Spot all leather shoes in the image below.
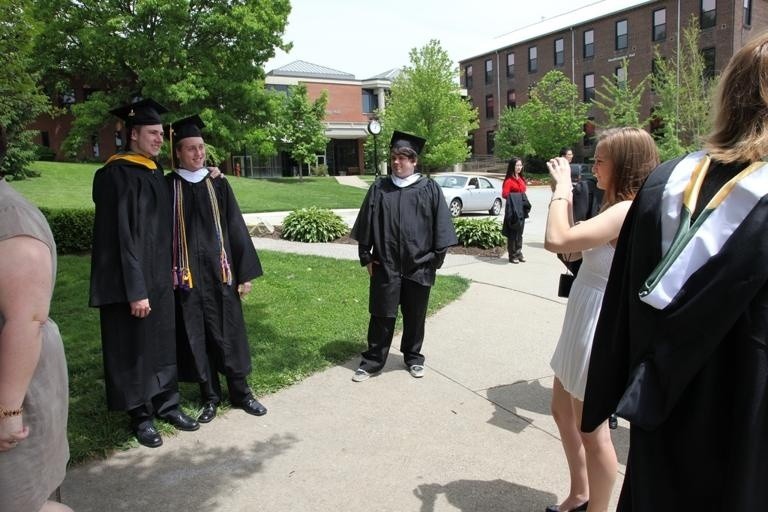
[546,501,588,512]
[136,422,161,447]
[199,403,219,421]
[165,409,199,430]
[243,397,267,416]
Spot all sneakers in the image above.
[352,367,382,382]
[409,364,424,378]
[509,258,525,263]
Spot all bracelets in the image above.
[0,407,23,418]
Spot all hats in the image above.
[108,98,169,126]
[162,114,207,172]
[387,130,426,155]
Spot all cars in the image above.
[426,171,504,219]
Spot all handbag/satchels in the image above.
[557,275,575,297]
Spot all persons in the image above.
[579,30,767,512]
[349,130,458,383]
[502,157,531,263]
[164,113,268,423]
[542,128,660,511]
[89,96,225,448]
[0,127,78,512]
